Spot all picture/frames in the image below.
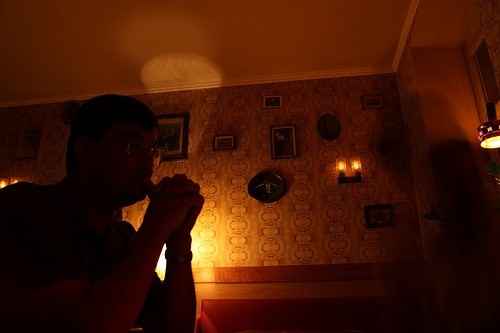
[361,93,384,109]
[263,94,282,109]
[213,133,236,151]
[14,123,47,166]
[364,203,395,230]
[270,124,298,160]
[153,112,190,162]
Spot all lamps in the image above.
[336,156,364,185]
[476,119,500,149]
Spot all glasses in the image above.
[104,136,160,158]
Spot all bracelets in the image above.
[163,249,193,264]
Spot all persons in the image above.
[1,94,204,332]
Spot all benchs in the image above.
[194,297,441,333]
